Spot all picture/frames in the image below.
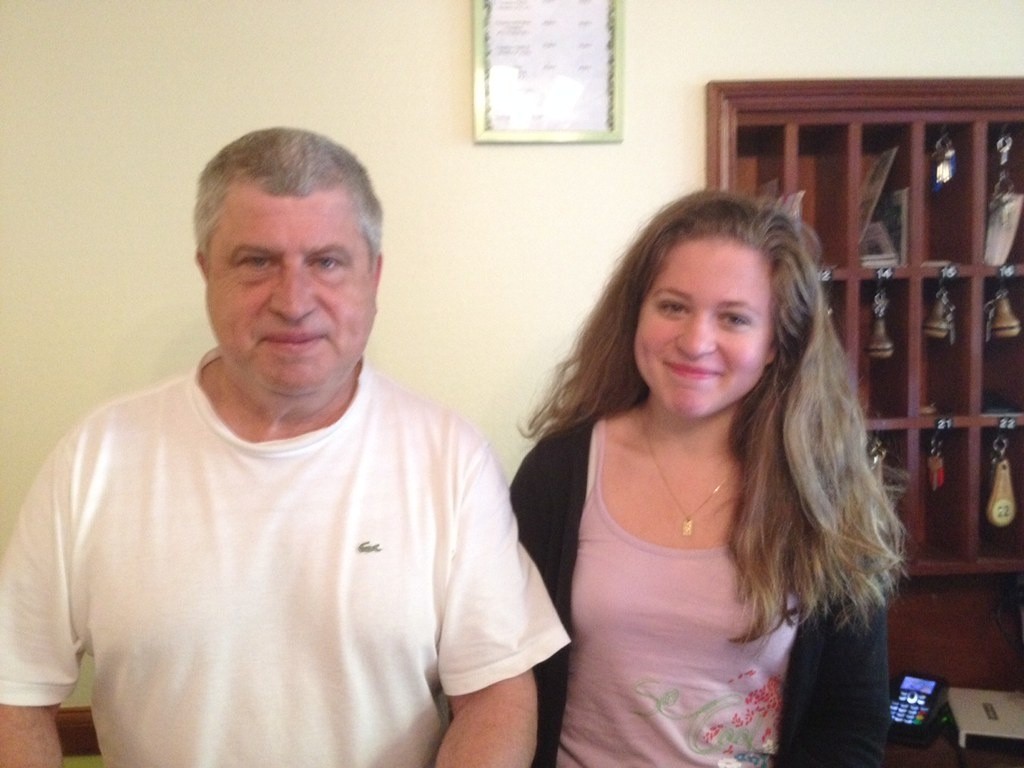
[471,0,627,142]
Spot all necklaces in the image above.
[640,413,735,539]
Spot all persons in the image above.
[0,128,572,768]
[506,188,910,768]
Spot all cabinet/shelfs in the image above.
[706,77,1023,583]
